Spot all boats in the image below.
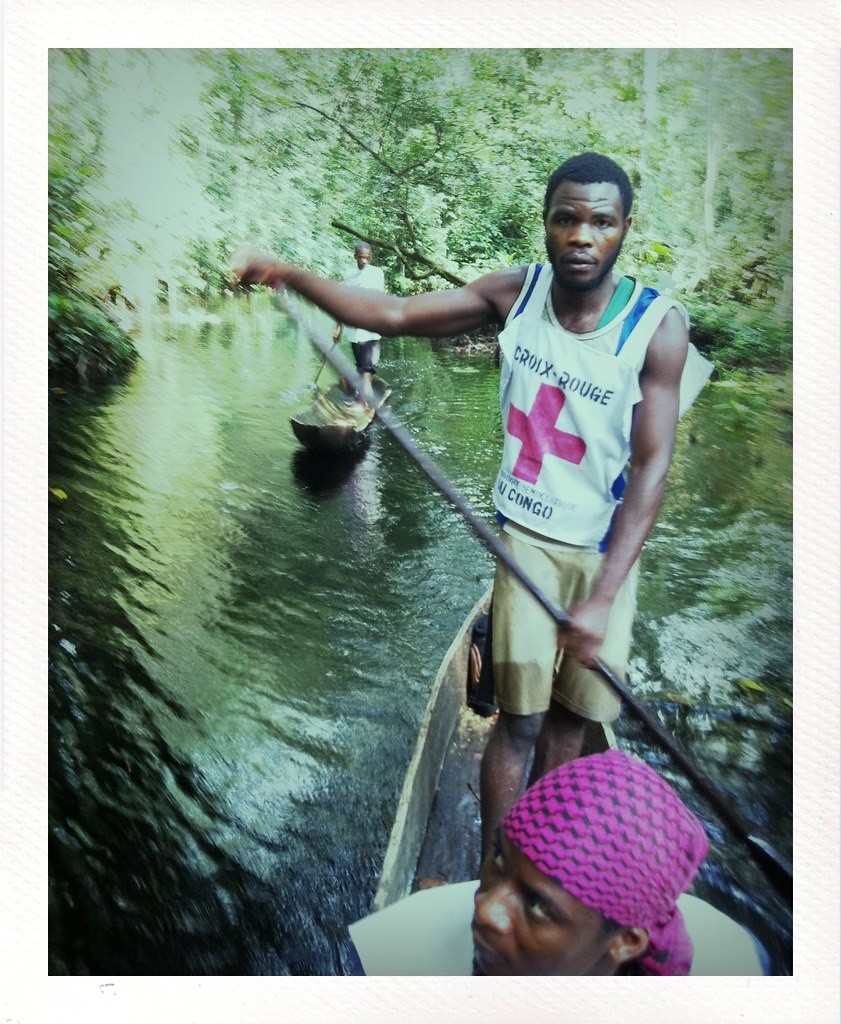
[289,371,397,458]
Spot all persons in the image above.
[348,750,772,976]
[235,152,688,884]
[331,242,384,411]
